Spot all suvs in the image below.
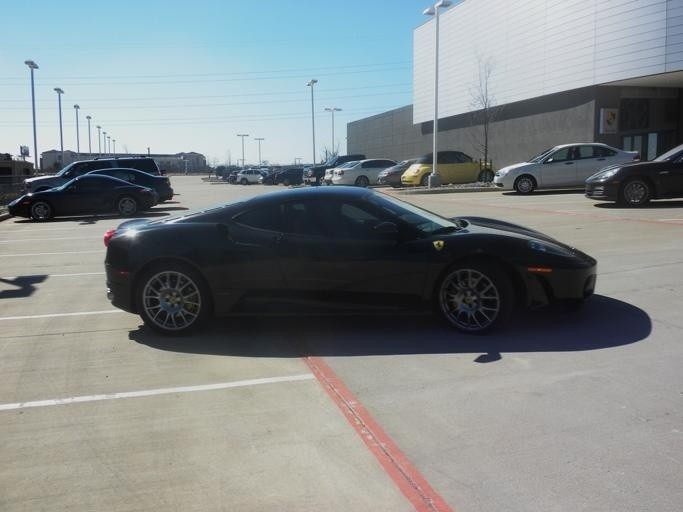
[21,154,161,196]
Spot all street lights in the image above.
[22,59,116,179]
[236,133,264,169]
[304,78,318,164]
[324,107,342,157]
[422,0,452,178]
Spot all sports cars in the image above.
[583,141,683,208]
[98,185,599,335]
[6,172,158,221]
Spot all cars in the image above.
[492,141,640,195]
[86,167,172,204]
[227,151,498,190]
